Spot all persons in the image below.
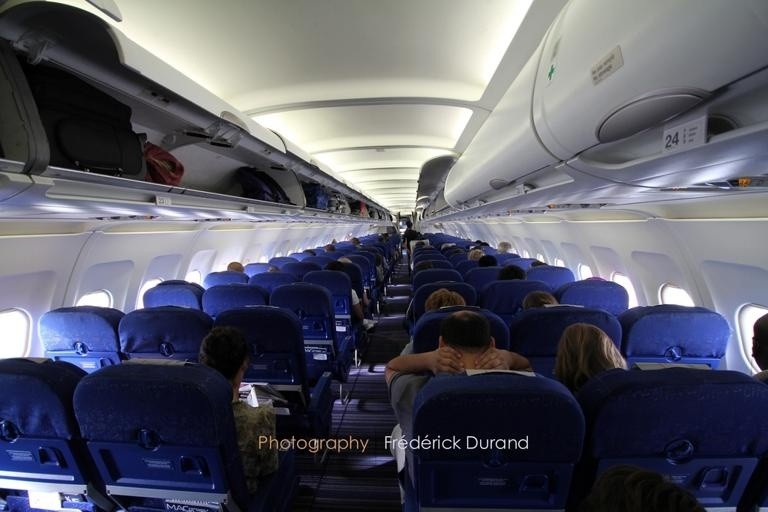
[553,322,627,392]
[196,218,625,354]
[382,310,533,504]
[750,311,768,384]
[198,327,279,495]
[568,462,708,511]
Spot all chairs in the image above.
[2,232,768,512]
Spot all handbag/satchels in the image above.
[301,181,385,220]
[18,54,183,186]
[237,167,290,203]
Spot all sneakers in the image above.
[363,318,378,330]
